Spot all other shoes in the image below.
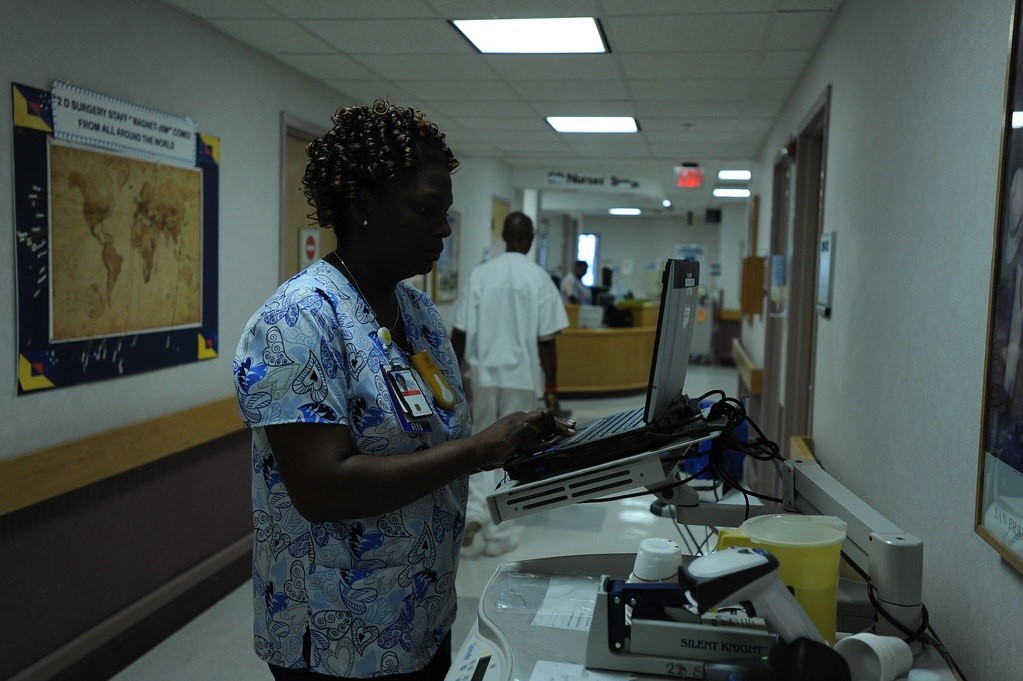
[484,531,520,556]
[461,517,482,547]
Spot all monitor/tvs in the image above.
[598,294,615,323]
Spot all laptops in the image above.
[504,258,701,481]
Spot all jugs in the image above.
[712,514,846,646]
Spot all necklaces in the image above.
[333,249,399,331]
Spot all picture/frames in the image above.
[973,0,1023,576]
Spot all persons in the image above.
[559,261,593,305]
[450,211,571,557]
[395,373,410,392]
[233,98,577,681]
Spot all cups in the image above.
[833,633,913,681]
[629,538,682,583]
